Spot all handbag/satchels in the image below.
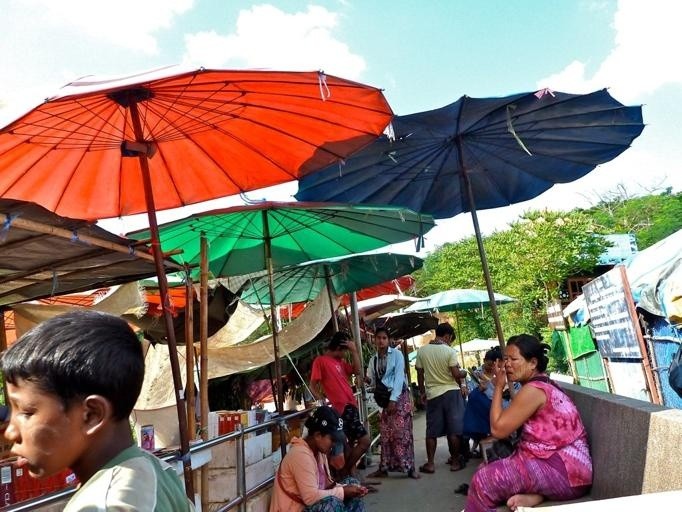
[373,381,391,409]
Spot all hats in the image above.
[304,405,348,443]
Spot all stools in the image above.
[479,438,494,464]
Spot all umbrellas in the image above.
[238,248,426,306]
[294,84,649,401]
[272,272,416,320]
[126,199,438,458]
[341,294,431,324]
[0,61,398,505]
[371,313,440,386]
[404,289,519,385]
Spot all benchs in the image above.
[533,379,682,510]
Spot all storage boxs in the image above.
[197,431,277,512]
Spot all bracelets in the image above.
[461,383,466,388]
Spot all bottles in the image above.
[351,374,356,385]
[216,411,248,436]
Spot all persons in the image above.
[308,329,380,494]
[462,349,496,440]
[0,307,205,512]
[362,328,420,480]
[267,404,369,512]
[462,332,594,512]
[414,322,468,473]
[479,347,523,411]
[447,332,471,463]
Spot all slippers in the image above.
[419,462,435,474]
[367,470,389,478]
[408,471,421,480]
[450,461,466,471]
